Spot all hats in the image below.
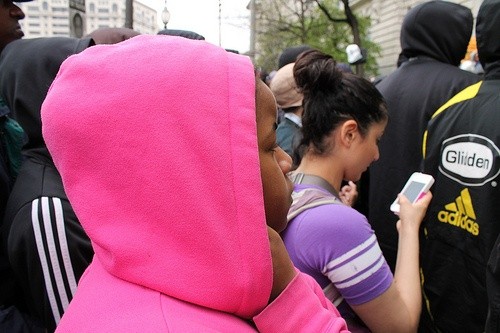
[345,43,363,64]
[270,63,304,109]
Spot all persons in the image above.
[39,33,351,333]
[352,0,481,280]
[421,0,500,333]
[279,49,433,333]
[0,0,484,332]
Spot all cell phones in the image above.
[390,172,435,214]
